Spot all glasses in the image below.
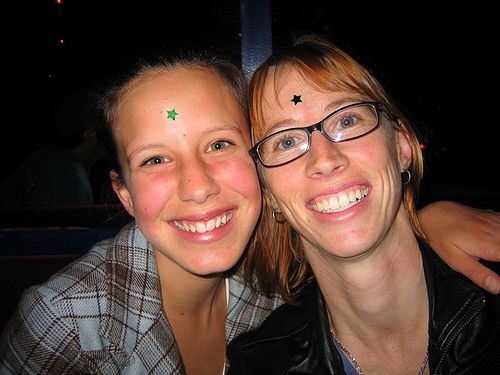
[247,102,398,168]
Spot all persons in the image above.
[0,43,500,375]
[214,35,500,375]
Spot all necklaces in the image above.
[331,330,427,374]
